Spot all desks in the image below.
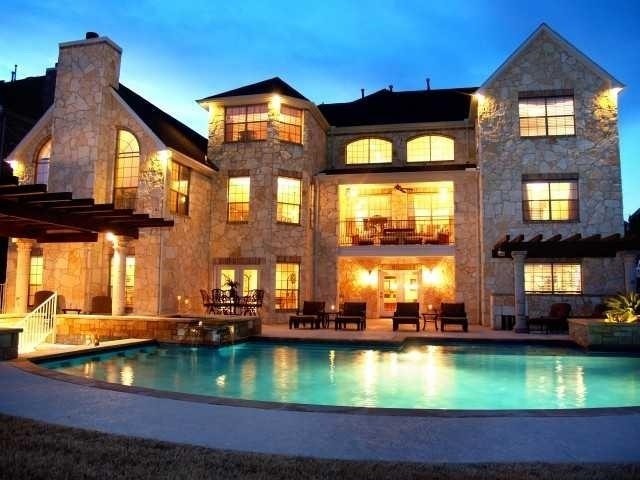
[61,308,81,314]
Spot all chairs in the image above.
[33,290,54,311]
[288,300,367,331]
[351,228,450,245]
[91,295,112,314]
[525,303,611,335]
[391,302,468,333]
[199,288,264,316]
